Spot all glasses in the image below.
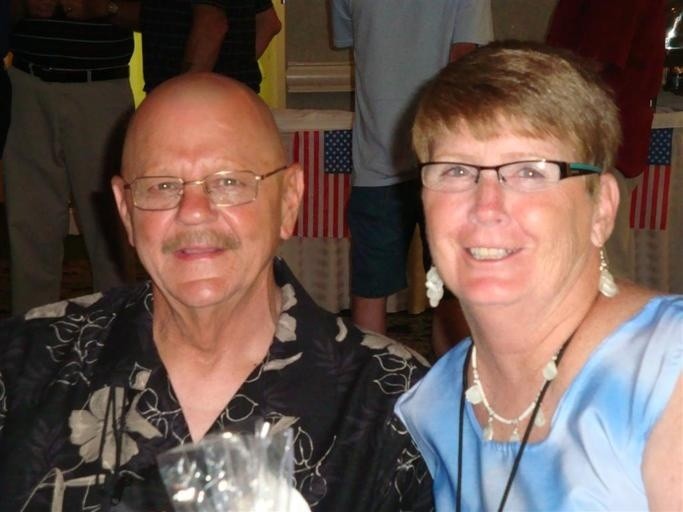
[416,159,602,190]
[124,165,287,212]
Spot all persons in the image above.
[392,39,682,509]
[0,1,140,310]
[1,69,434,511]
[327,1,499,363]
[539,2,669,285]
[136,1,283,99]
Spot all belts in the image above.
[11,60,130,83]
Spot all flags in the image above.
[627,128,672,229]
[280,129,352,240]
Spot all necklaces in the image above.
[465,346,556,445]
